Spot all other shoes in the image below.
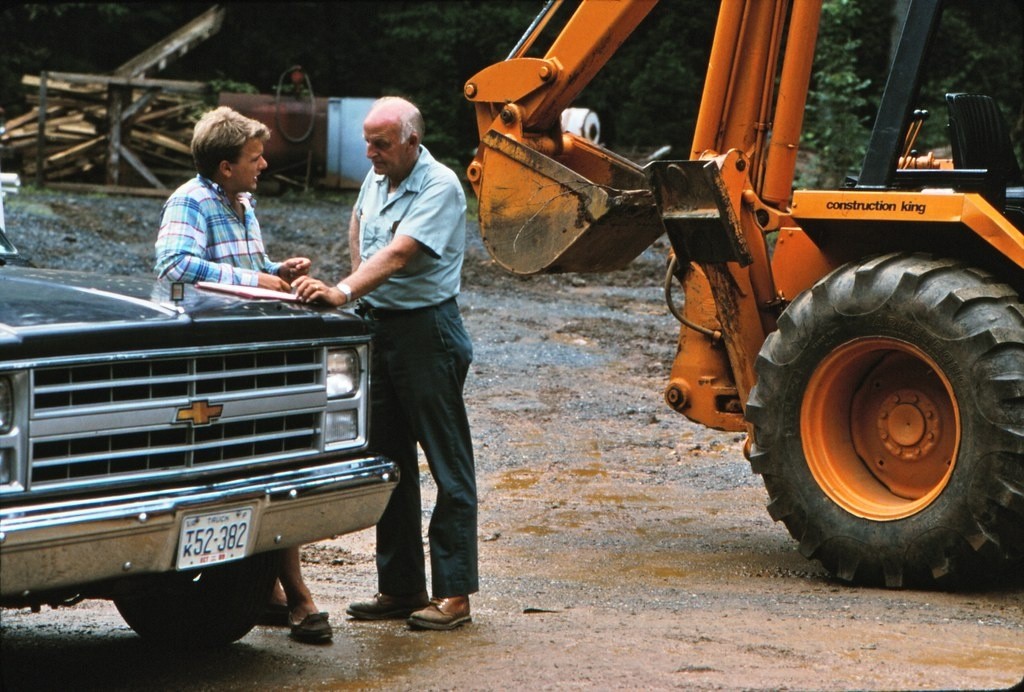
[286,609,333,641]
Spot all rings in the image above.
[314,283,319,291]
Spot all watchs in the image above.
[338,283,351,304]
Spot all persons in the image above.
[154,106,333,641]
[291,96,480,630]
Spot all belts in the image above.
[365,308,395,320]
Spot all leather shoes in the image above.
[346,592,430,619]
[408,595,472,629]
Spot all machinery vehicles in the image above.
[461,0,1024,591]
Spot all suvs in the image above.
[0,220,402,651]
